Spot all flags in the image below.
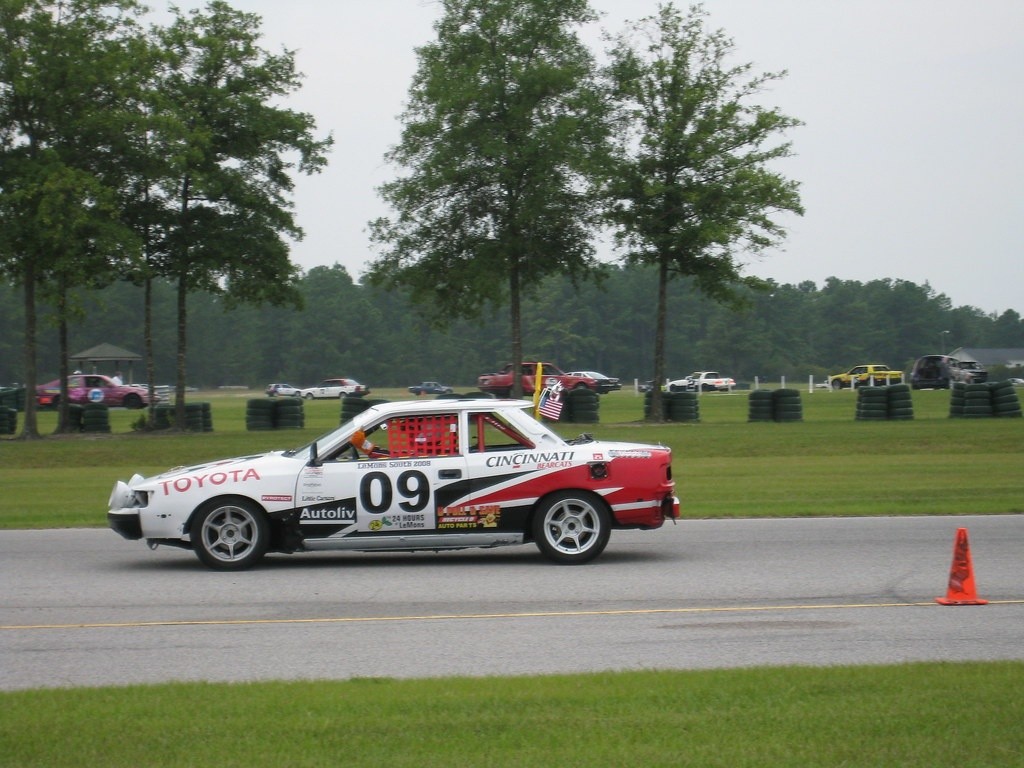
[537,396,563,420]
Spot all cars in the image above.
[478,362,601,398]
[688,371,737,392]
[300,378,374,396]
[909,353,989,391]
[565,370,623,395]
[409,382,455,396]
[830,365,904,390]
[266,383,303,398]
[35,374,149,411]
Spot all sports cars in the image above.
[105,395,680,571]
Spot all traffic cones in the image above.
[936,527,992,606]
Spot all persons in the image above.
[350,418,444,457]
[112,371,123,385]
